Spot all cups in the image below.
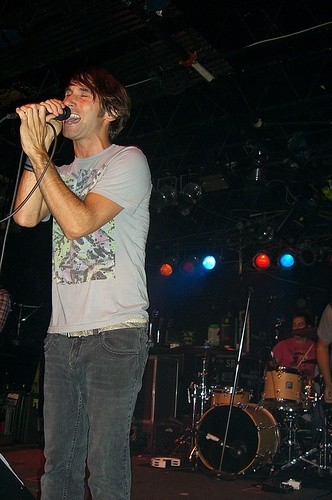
[154,328,162,344]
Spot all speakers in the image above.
[131,355,180,425]
[0,453,37,500]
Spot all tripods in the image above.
[160,416,236,480]
[267,397,332,483]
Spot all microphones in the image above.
[234,447,247,456]
[271,351,278,367]
[8,106,71,121]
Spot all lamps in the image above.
[152,175,176,207]
[179,182,202,204]
[252,250,272,271]
[277,251,297,271]
[216,136,289,188]
[180,255,198,275]
[159,248,177,276]
[200,247,223,270]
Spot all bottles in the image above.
[220,318,233,346]
[208,324,220,346]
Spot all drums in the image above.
[261,365,305,405]
[194,402,281,480]
[210,386,250,408]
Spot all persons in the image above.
[0,288,11,330]
[13,66,152,500]
[267,308,317,385]
[317,299,332,404]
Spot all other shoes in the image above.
[282,479,300,490]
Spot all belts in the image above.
[59,319,147,337]
